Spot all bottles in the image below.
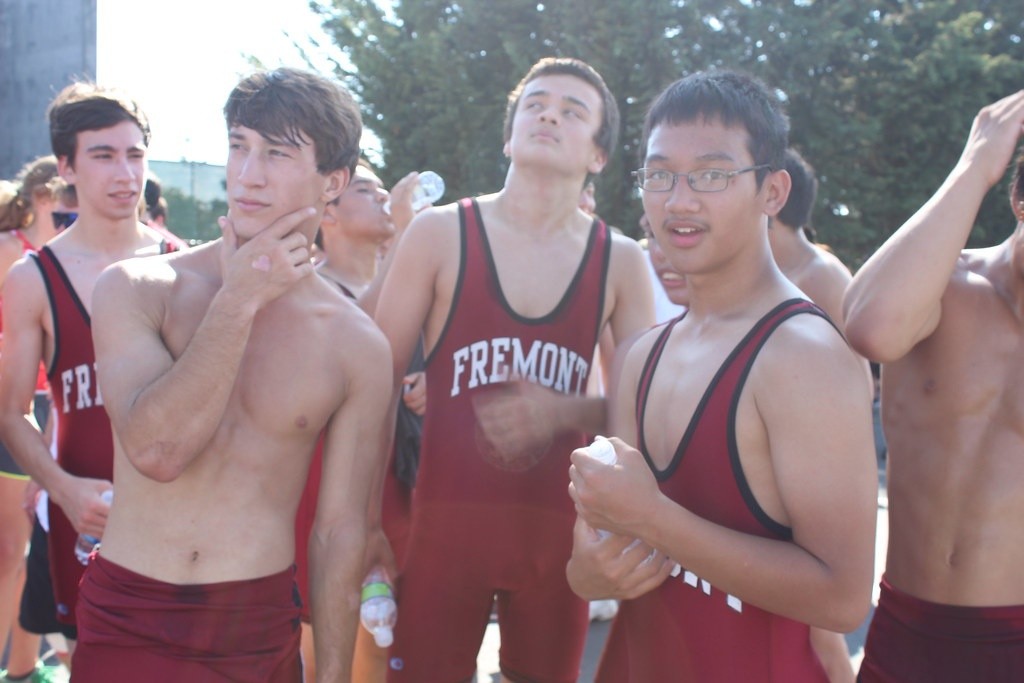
[359,563,397,647]
[384,171,446,216]
[74,487,116,565]
[573,438,672,571]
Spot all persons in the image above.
[574,180,620,396]
[766,147,877,406]
[0,180,26,232]
[0,155,68,683]
[292,155,435,683]
[137,170,831,329]
[564,71,880,683]
[840,87,1024,683]
[368,57,658,683]
[634,202,858,682]
[0,81,183,628]
[68,68,394,682]
[19,176,88,683]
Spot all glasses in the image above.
[631,162,773,192]
